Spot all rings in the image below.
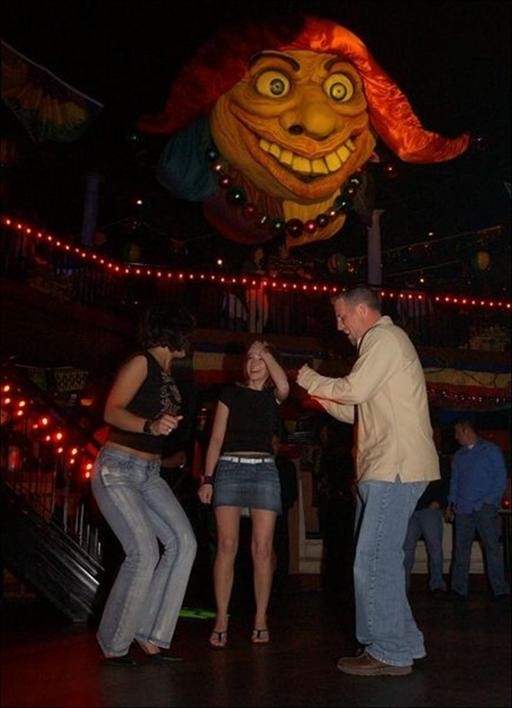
[166,427,172,431]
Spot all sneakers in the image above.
[428,584,468,602]
[335,647,413,677]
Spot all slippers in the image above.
[251,624,269,645]
[208,629,228,651]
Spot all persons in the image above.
[293,284,441,676]
[10,212,57,288]
[159,429,202,608]
[195,334,290,651]
[269,240,314,333]
[443,414,511,605]
[240,243,270,333]
[87,300,202,671]
[78,227,119,307]
[270,428,299,595]
[403,418,449,598]
[307,417,355,600]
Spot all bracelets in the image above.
[200,474,213,485]
[143,419,153,436]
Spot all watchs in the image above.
[259,345,271,357]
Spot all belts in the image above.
[219,455,275,465]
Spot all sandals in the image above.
[130,627,183,662]
[102,654,146,678]
